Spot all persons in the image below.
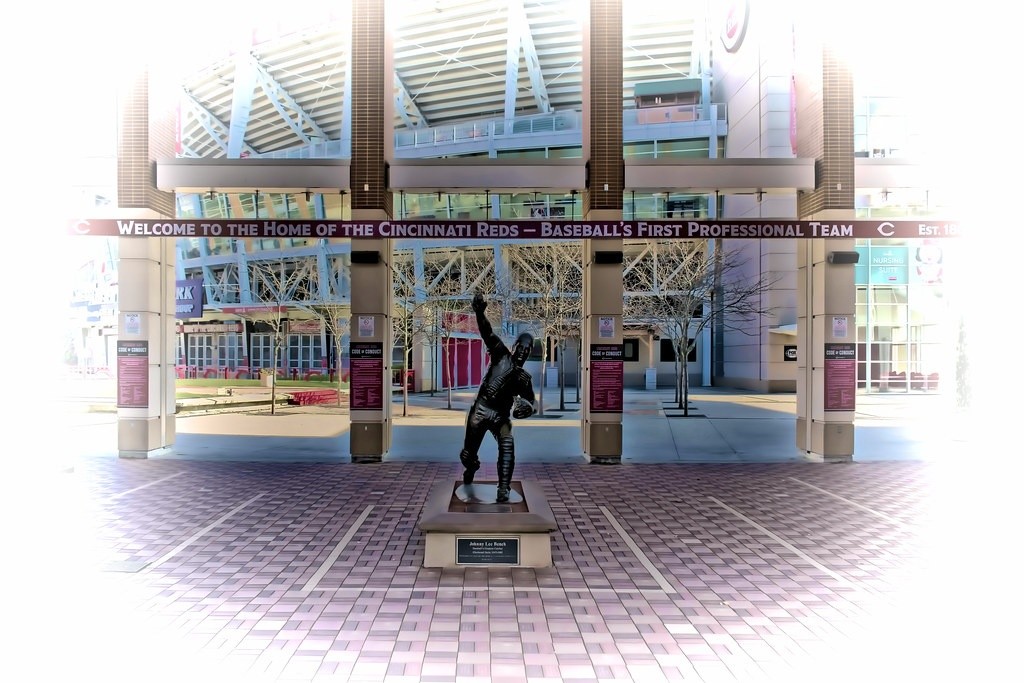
[460,293,538,501]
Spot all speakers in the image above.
[829,252,859,264]
[595,251,623,264]
[350,251,379,263]
